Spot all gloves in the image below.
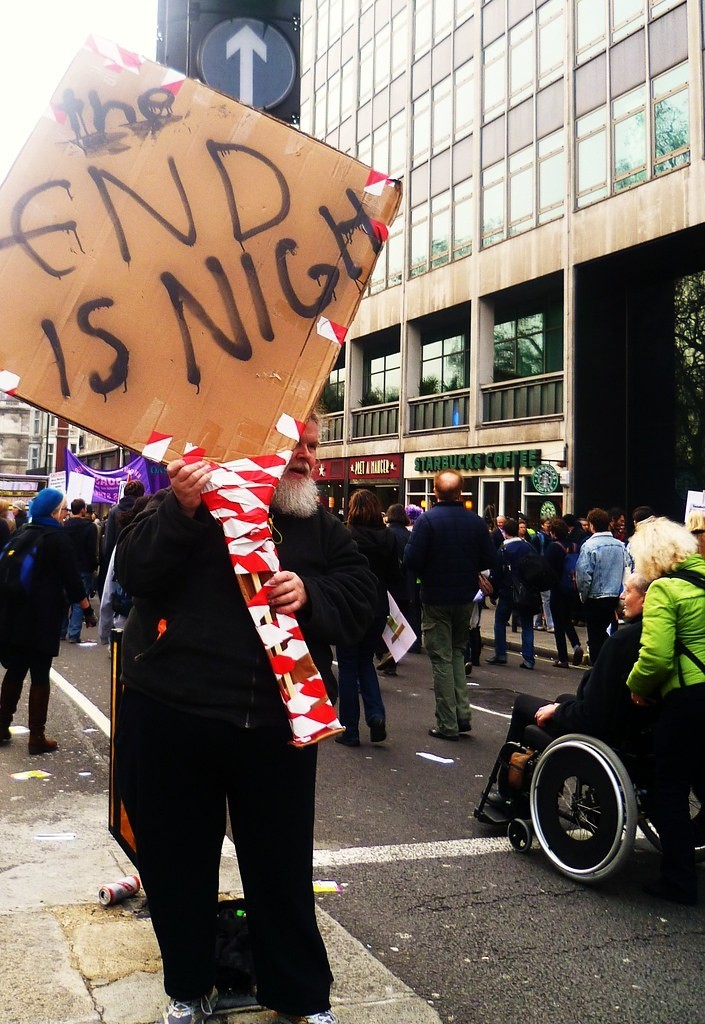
[83,605,97,628]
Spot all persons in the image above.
[400,469,500,736]
[113,400,390,1024]
[510,569,661,793]
[0,479,154,757]
[460,504,653,675]
[316,485,425,749]
[626,516,704,854]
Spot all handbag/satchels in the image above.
[512,583,542,611]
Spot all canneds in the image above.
[99,875,141,905]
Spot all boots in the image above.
[0,681,22,741]
[29,685,57,755]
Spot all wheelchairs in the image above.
[473,690,705,887]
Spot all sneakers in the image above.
[163,997,213,1024]
[278,1009,339,1024]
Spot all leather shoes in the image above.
[482,787,505,806]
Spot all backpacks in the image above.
[0,529,53,601]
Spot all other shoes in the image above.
[458,720,472,732]
[335,734,359,746]
[370,717,386,742]
[376,652,394,670]
[553,660,569,668]
[428,728,458,740]
[465,661,472,675]
[641,870,698,904]
[573,647,584,666]
[70,638,81,642]
[485,657,506,663]
[520,662,527,668]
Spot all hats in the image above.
[29,488,63,516]
